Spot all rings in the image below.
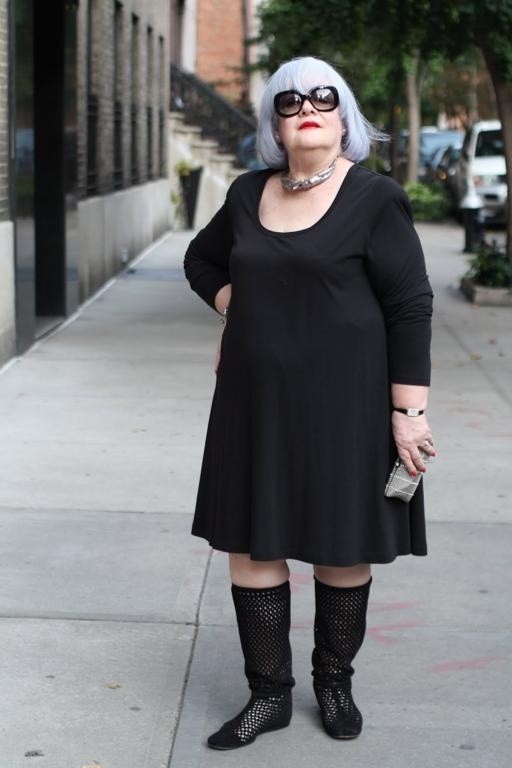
[421,439,430,450]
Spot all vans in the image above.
[450,118,509,229]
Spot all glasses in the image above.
[273,87,337,118]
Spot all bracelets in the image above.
[221,307,228,322]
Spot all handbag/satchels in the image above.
[385,448,429,504]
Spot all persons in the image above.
[183,55,435,749]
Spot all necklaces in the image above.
[278,159,338,190]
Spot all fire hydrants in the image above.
[458,194,487,251]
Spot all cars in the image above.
[425,146,464,199]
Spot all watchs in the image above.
[396,408,425,416]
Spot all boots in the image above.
[208,582,296,750]
[313,580,372,741]
